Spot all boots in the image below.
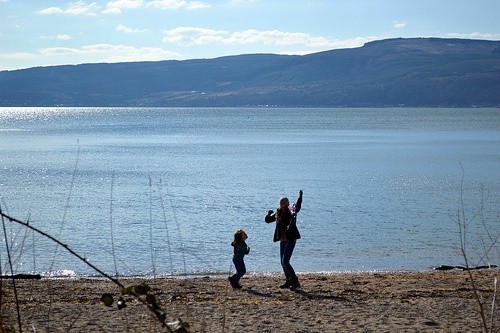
[290,276,300,290]
[280,279,292,288]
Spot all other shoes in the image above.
[235,284,242,289]
[228,277,235,288]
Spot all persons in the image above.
[228,229,250,290]
[265,189,304,291]
